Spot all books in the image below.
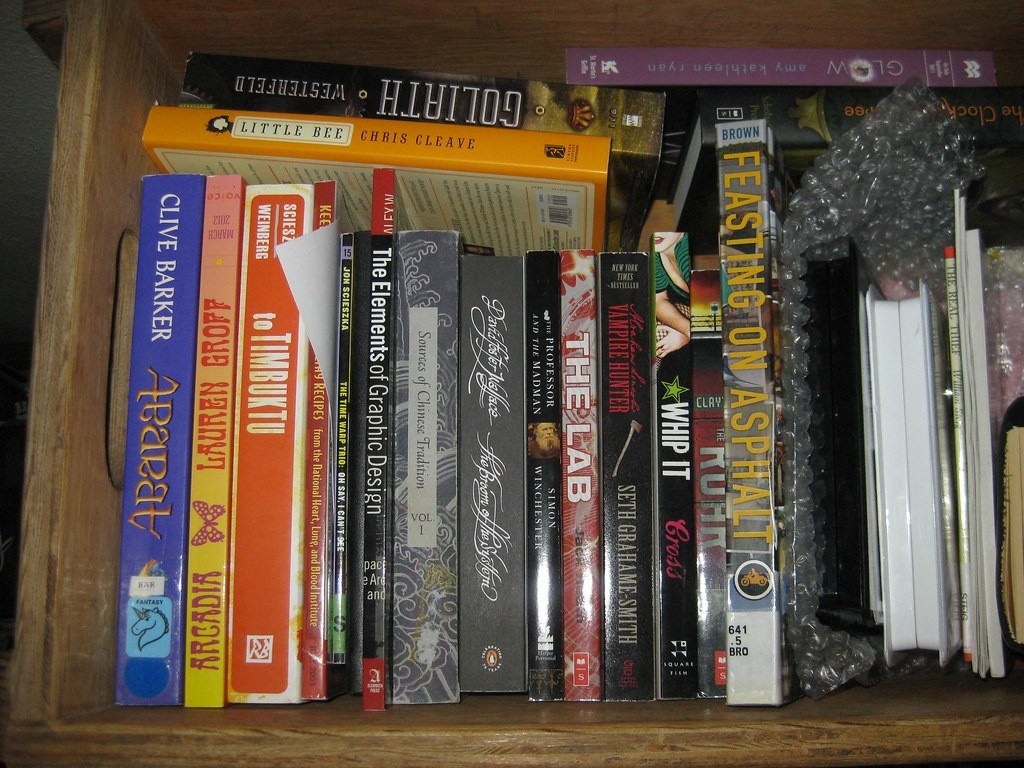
[115,47,1024,709]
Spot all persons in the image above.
[527,422,560,459]
[653,232,691,358]
